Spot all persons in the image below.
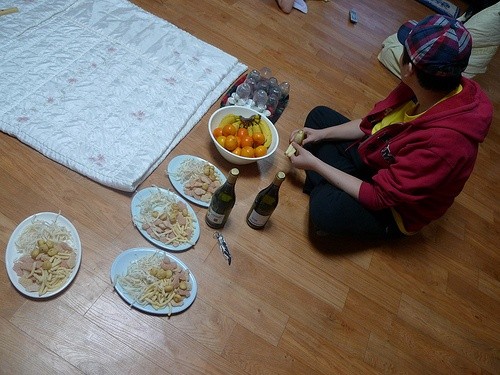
[289,14,493,256]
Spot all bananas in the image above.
[284,130,305,157]
[219,114,272,149]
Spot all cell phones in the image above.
[350,9,357,22]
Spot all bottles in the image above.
[225,69,291,118]
[205,168,239,229]
[246,171,286,230]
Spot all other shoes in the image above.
[277,0,294,14]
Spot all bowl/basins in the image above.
[208,105,279,165]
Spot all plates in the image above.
[111,247,198,315]
[130,188,200,251]
[5,211,81,298]
[167,154,226,208]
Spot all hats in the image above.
[397,15,473,77]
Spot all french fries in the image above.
[27,251,72,296]
[177,159,207,184]
[145,209,197,245]
[140,277,177,317]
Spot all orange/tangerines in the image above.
[213,125,267,158]
